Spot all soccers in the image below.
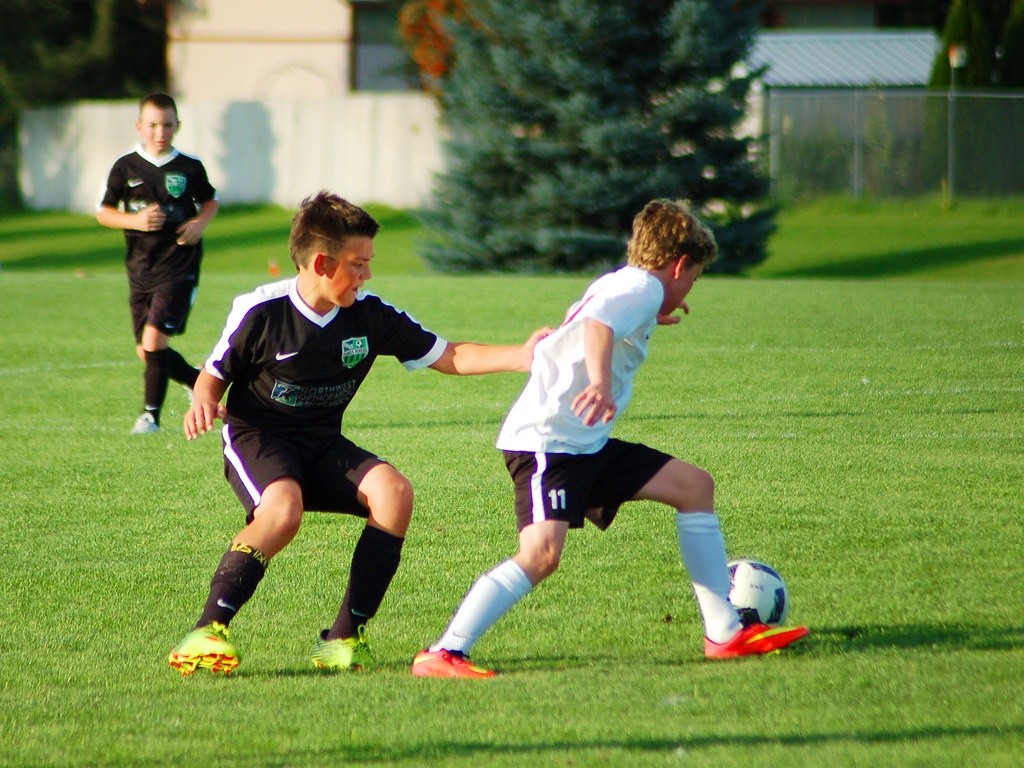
[726,559,788,626]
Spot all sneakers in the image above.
[310,629,380,673]
[169,621,239,677]
[412,648,497,679]
[704,623,809,659]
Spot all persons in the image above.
[411,198,810,679]
[165,190,557,677]
[94,92,222,434]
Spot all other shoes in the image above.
[131,415,161,434]
[185,366,205,407]
